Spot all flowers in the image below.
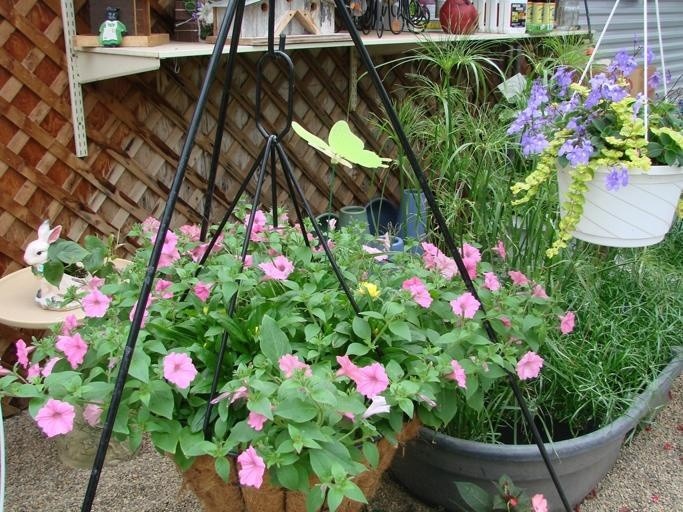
[197,4,215,26]
[1,199,573,512]
[501,42,683,256]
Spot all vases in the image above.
[310,189,425,252]
[164,412,418,512]
[551,149,683,249]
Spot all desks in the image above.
[0,259,147,468]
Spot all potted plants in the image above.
[388,20,683,508]
